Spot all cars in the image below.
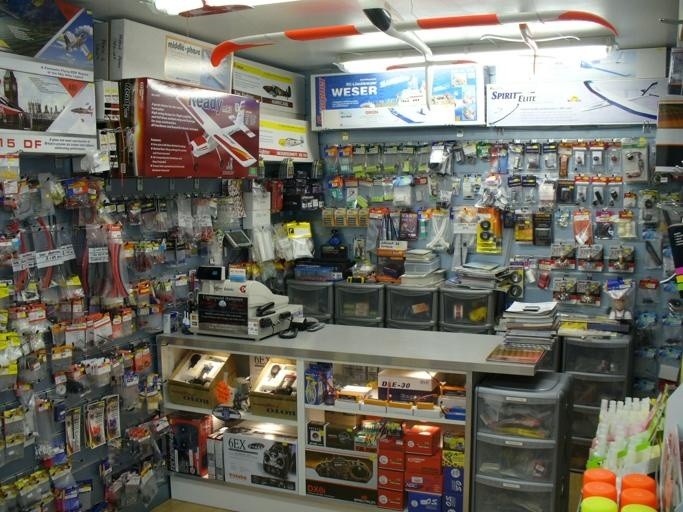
[0,95,25,125]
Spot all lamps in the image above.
[141,0,254,18]
[333,34,615,77]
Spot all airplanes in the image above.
[263,83,293,101]
[60,26,92,60]
[176,93,257,172]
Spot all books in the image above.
[486,299,558,364]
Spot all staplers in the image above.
[256,302,275,317]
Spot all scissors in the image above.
[186,287,199,311]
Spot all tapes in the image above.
[510,271,522,297]
[55,385,66,395]
[480,220,490,240]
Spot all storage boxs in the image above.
[561,334,633,471]
[441,279,494,328]
[472,372,577,510]
[306,361,464,512]
[387,280,439,326]
[335,278,383,322]
[161,340,296,496]
[287,279,332,319]
